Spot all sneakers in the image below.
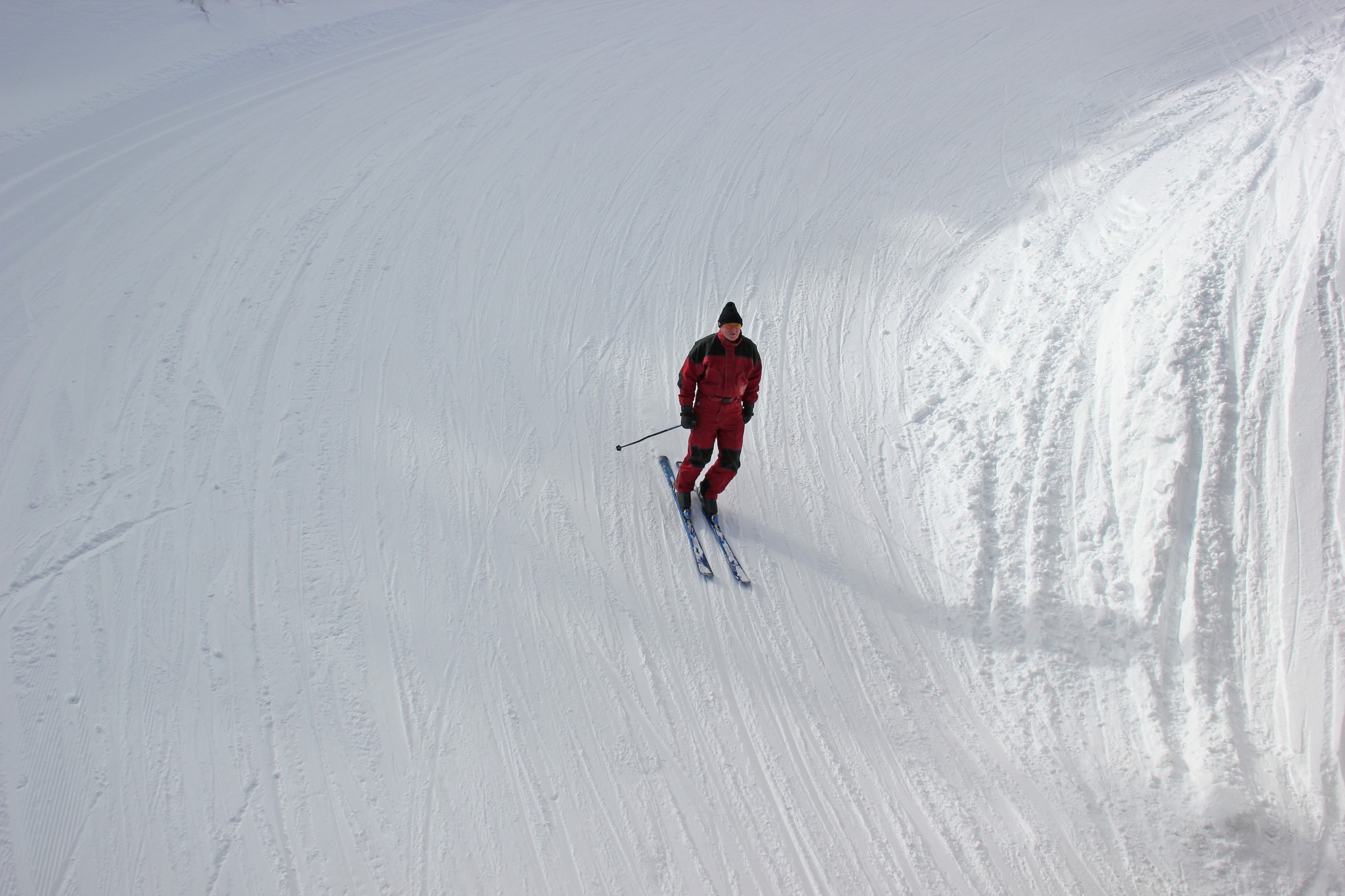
[677,492,691,510]
[700,483,718,515]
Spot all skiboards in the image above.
[657,454,753,585]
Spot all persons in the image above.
[673,302,762,515]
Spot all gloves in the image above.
[680,406,697,429]
[742,402,755,424]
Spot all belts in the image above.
[708,395,736,405]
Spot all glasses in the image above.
[723,323,741,329]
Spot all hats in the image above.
[718,302,743,328]
